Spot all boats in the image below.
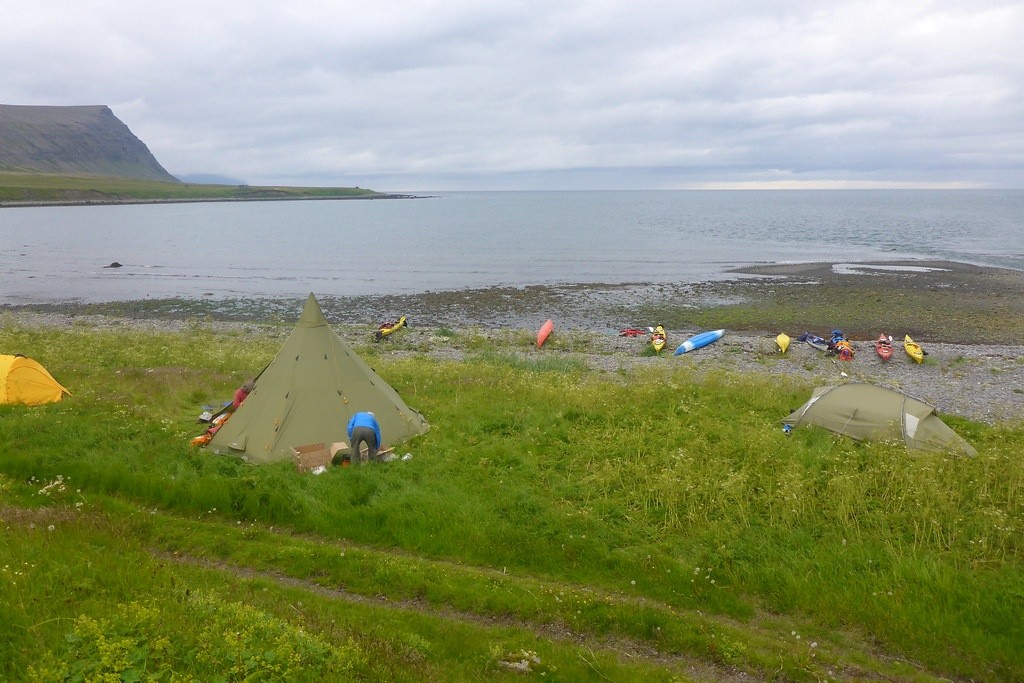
[904,335,923,364]
[805,331,829,351]
[876,333,893,360]
[371,317,406,343]
[537,319,553,349]
[776,333,790,354]
[652,326,667,353]
[673,329,725,356]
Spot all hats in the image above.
[245,381,254,392]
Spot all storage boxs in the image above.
[291,441,332,474]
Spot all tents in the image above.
[781,380,978,459]
[207,293,428,467]
[0,354,71,406]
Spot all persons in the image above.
[347,411,381,466]
